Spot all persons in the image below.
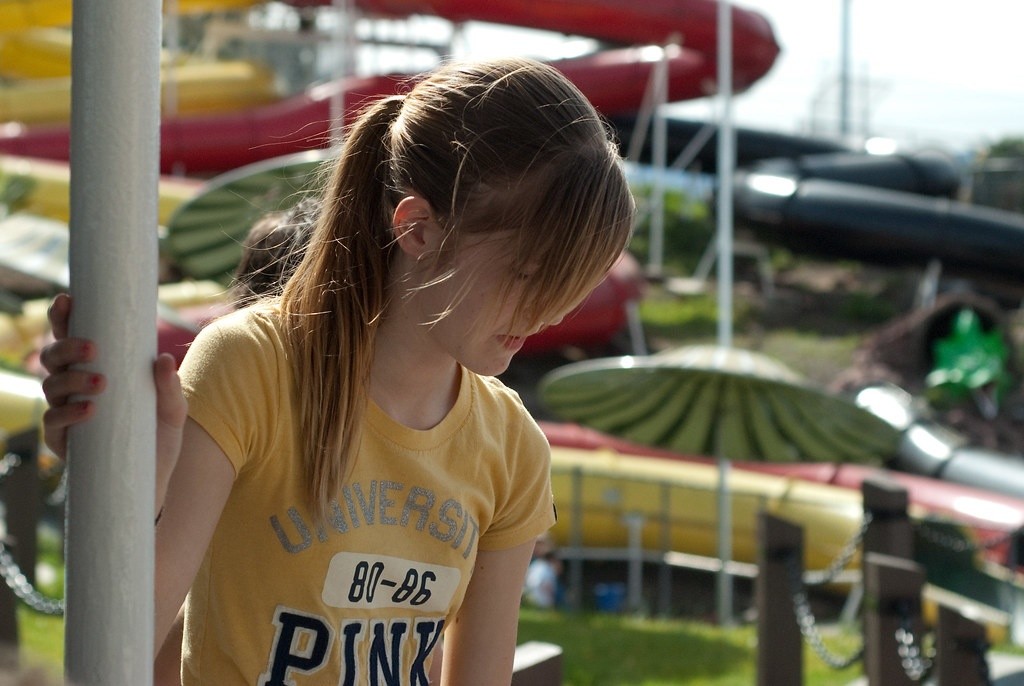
[39,58,634,686]
[834,296,1009,454]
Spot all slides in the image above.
[0,0,938,590]
